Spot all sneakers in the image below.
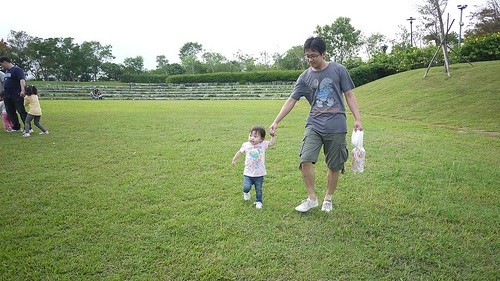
[295,197,318,212]
[322,197,332,212]
[243,192,251,200]
[256,202,262,209]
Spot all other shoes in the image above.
[23,133,30,137]
[11,128,23,132]
[5,130,12,133]
[39,131,49,135]
[22,129,33,133]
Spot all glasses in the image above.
[304,53,321,59]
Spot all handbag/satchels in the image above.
[351,128,365,174]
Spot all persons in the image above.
[269,36,363,212]
[22,85,50,137]
[0,56,33,133]
[93,89,102,99]
[232,125,276,209]
